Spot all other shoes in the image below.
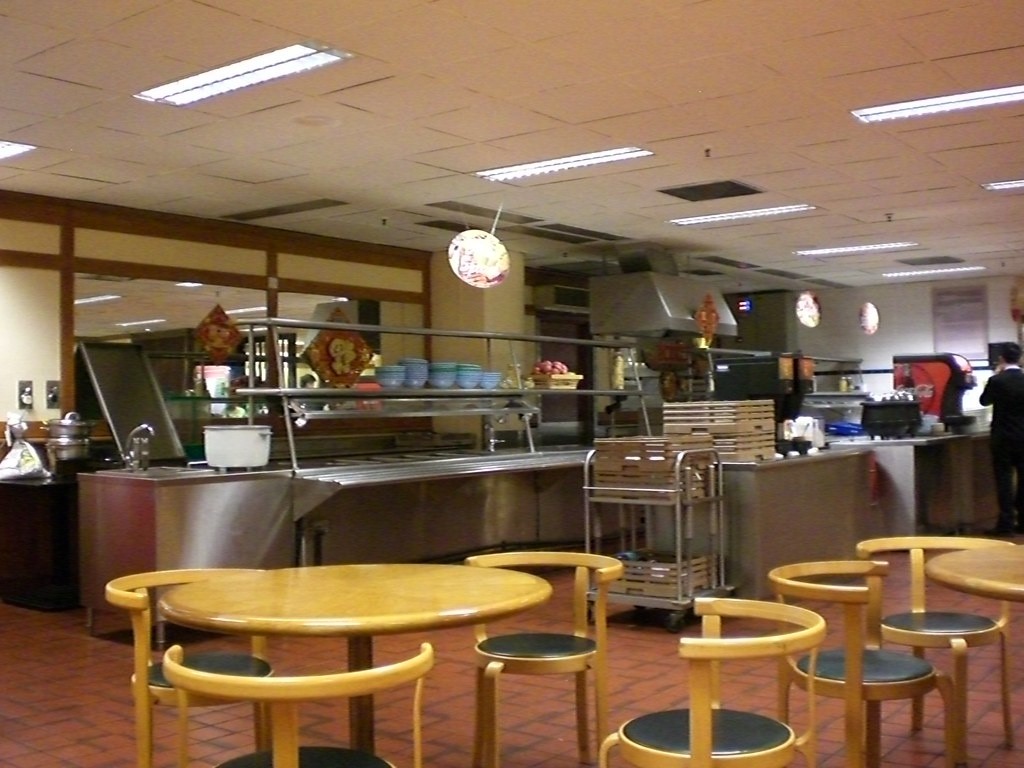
[984,528,1013,537]
[1013,525,1024,534]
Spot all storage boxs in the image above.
[593,433,714,498]
[662,398,778,462]
[607,551,718,596]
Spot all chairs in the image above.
[106,536,1024,768]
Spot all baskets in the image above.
[520,374,584,389]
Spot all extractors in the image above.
[585,267,739,341]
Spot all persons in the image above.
[979,341,1024,537]
[47,386,60,408]
[20,390,32,408]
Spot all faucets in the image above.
[125,422,159,451]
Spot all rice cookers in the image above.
[201,424,272,472]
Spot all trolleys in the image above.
[581,450,739,634]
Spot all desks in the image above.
[926,543,1024,604]
[158,564,553,755]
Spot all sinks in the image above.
[95,462,215,478]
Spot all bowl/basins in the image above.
[919,414,977,436]
[375,359,503,389]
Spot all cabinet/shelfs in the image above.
[236,318,654,476]
[584,448,728,628]
